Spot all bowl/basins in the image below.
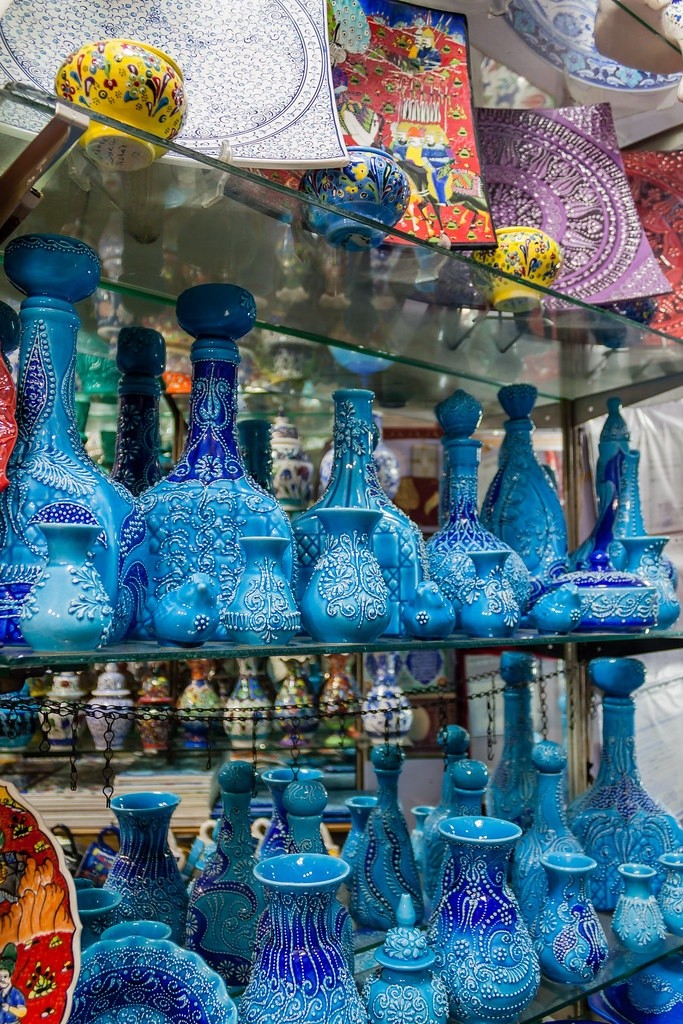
[545,550,659,633]
[471,227,563,313]
[55,38,187,172]
[298,146,411,251]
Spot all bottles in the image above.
[71,744,451,1024]
[425,651,683,1024]
[0,651,418,749]
[1,233,681,652]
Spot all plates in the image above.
[0,779,83,1024]
[494,0,683,92]
[222,0,499,251]
[67,935,238,1024]
[0,0,350,169]
[471,103,683,348]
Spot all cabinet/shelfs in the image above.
[0,0,683,1024]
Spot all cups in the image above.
[74,826,121,887]
[51,824,82,878]
[181,819,219,878]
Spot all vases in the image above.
[72,768,683,1024]
[619,535,681,630]
[302,507,392,645]
[468,225,566,312]
[177,651,414,752]
[300,148,410,252]
[17,508,116,657]
[221,537,302,647]
[58,41,187,172]
[461,550,520,639]
